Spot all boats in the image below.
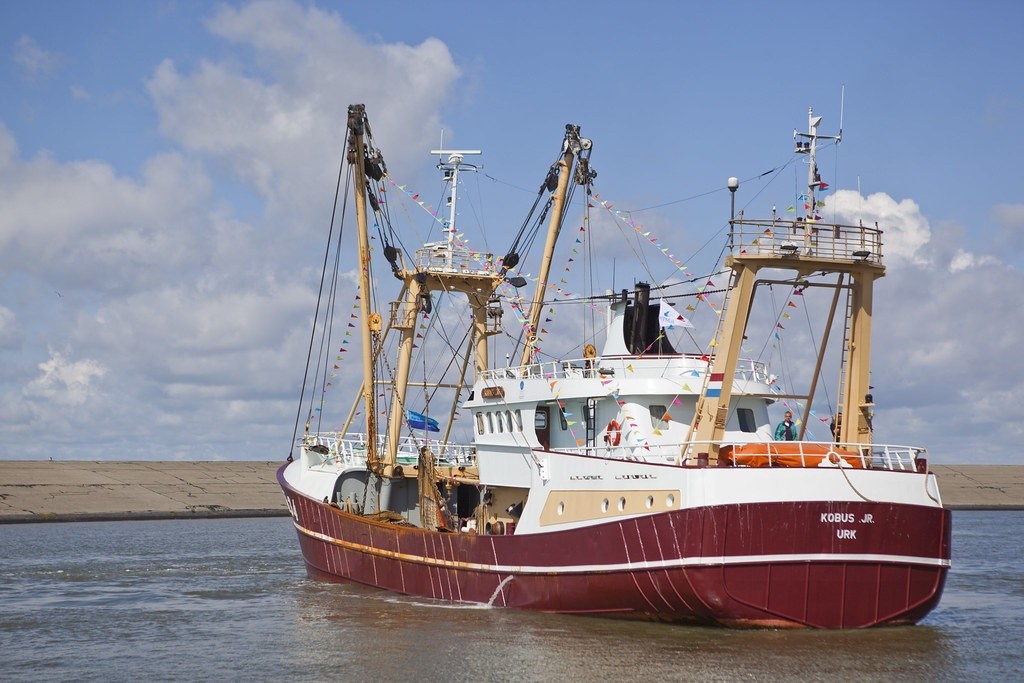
[276,101,953,631]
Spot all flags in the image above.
[409,412,440,432]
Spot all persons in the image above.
[775,411,798,441]
[830,412,842,448]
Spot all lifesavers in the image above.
[606,420,621,445]
